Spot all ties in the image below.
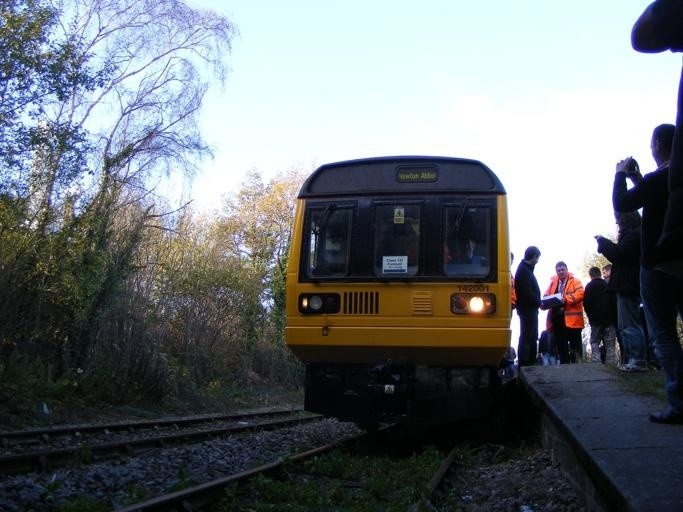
[560,281,564,293]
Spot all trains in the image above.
[284,155,521,443]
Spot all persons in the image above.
[637,300,662,371]
[538,260,587,365]
[610,121,683,429]
[510,252,517,309]
[601,263,629,365]
[537,327,577,366]
[513,246,543,367]
[583,266,619,368]
[627,0,683,255]
[445,235,488,273]
[594,208,650,375]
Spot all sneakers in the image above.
[649,405,683,424]
[618,359,649,372]
